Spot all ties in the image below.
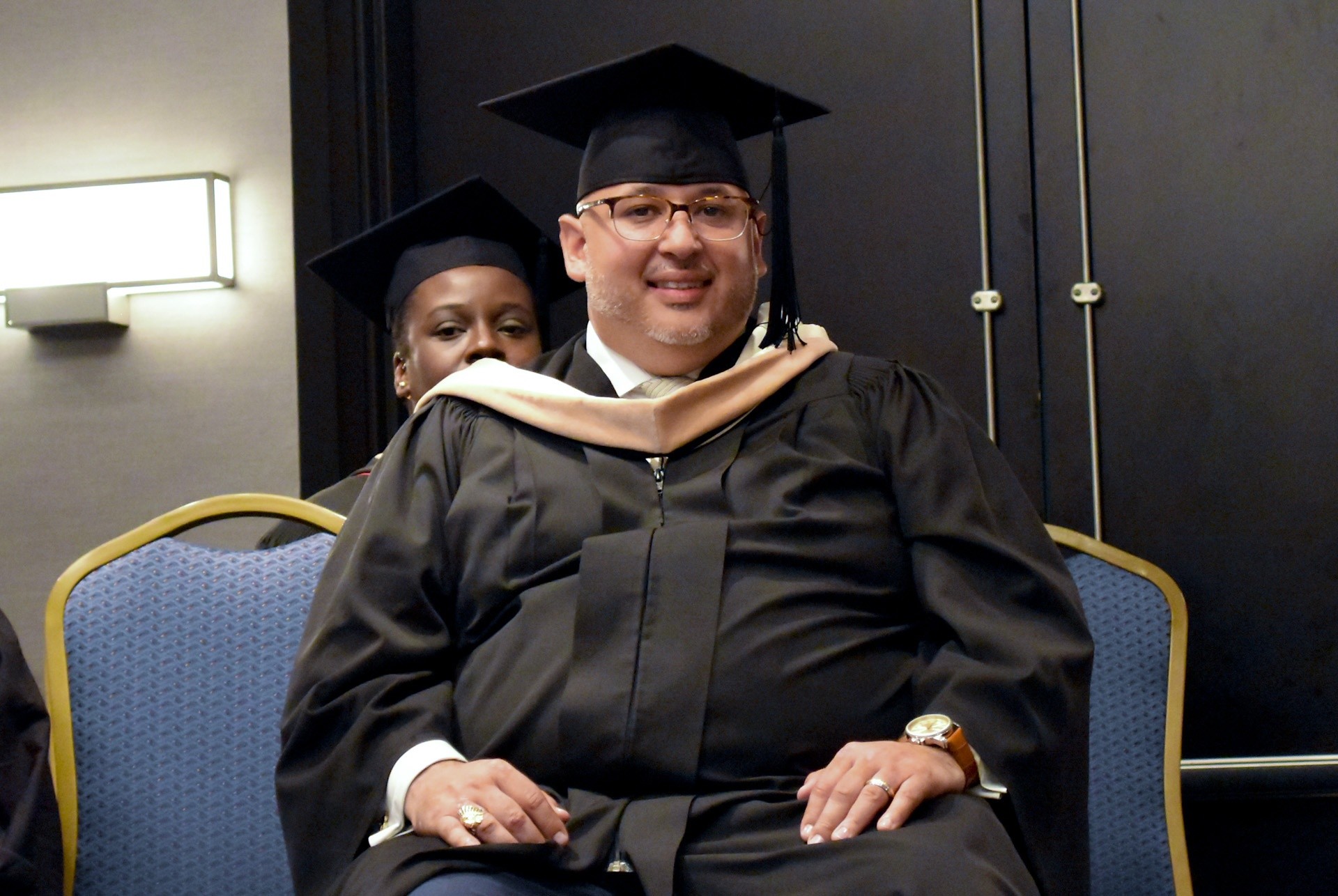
[628,375,697,398]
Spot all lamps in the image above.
[0,173,234,332]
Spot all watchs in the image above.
[899,712,979,793]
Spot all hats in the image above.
[307,173,590,337]
[479,43,832,356]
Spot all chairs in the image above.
[42,492,350,896]
[1040,523,1195,896]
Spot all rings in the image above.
[457,804,485,832]
[865,777,895,798]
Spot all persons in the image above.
[250,175,587,553]
[269,46,1094,896]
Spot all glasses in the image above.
[568,194,758,244]
